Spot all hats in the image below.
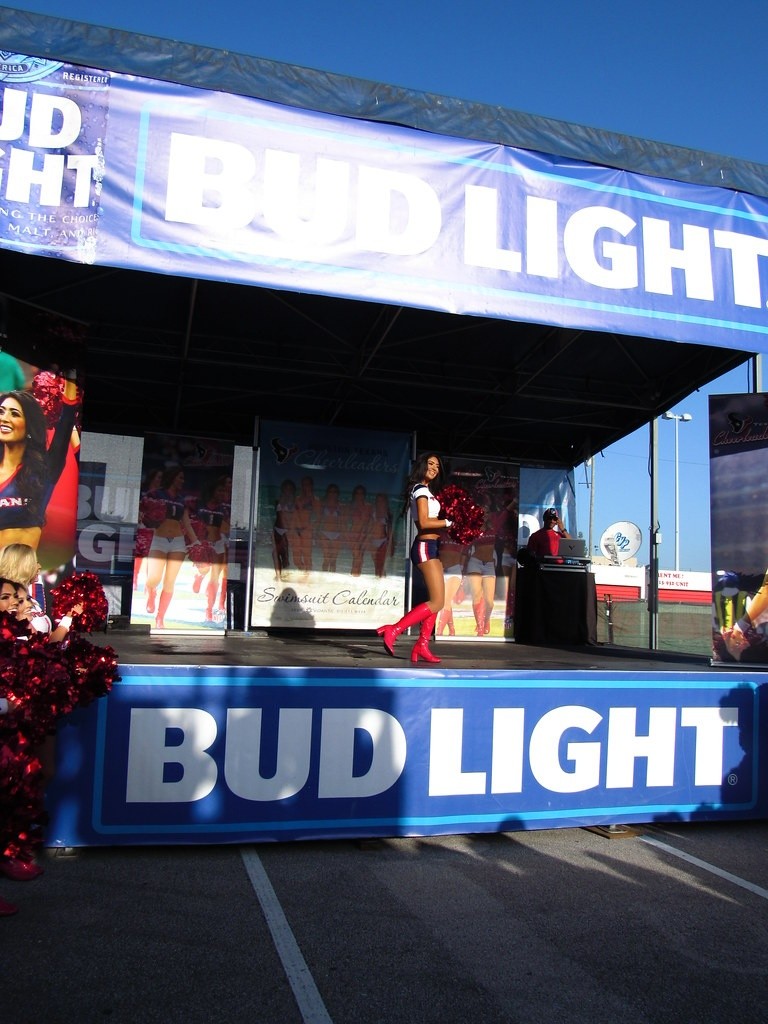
[543,508,559,519]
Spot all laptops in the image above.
[557,538,585,557]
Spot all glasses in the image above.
[546,517,558,521]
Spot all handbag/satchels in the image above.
[453,585,467,605]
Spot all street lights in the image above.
[660,411,694,570]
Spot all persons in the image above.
[0,543,85,918]
[137,465,234,563]
[440,477,519,631]
[527,508,572,564]
[0,350,80,587]
[376,452,456,664]
[730,563,768,643]
[267,478,395,575]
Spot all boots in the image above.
[146,583,157,614]
[410,611,442,663]
[205,583,220,621]
[219,577,227,613]
[483,602,495,634]
[375,602,433,656]
[155,590,175,629]
[472,597,486,637]
[193,573,204,593]
[132,558,143,590]
[447,610,456,636]
[436,609,451,636]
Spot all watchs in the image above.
[562,529,567,533]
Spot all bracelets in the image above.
[444,519,451,528]
